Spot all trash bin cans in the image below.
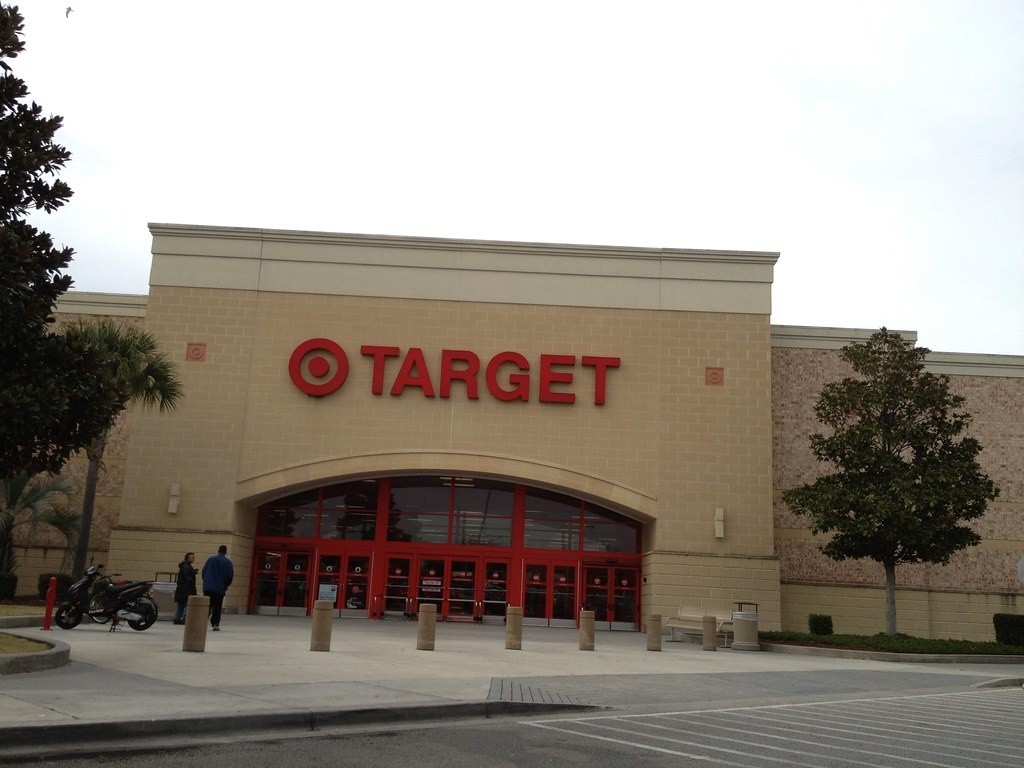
[731,612,761,651]
[152,581,177,621]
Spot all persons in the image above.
[174,552,200,625]
[202,545,234,632]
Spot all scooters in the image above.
[55,553,159,632]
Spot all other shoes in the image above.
[213,626,221,631]
[174,619,185,624]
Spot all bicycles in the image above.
[88,573,158,624]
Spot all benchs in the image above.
[664,605,735,649]
